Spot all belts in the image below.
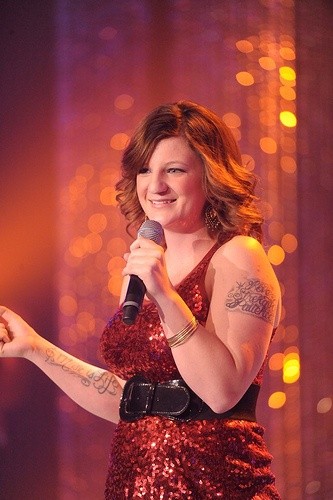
[117,379,261,425]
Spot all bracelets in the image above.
[165,316,201,350]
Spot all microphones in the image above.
[123,220,163,325]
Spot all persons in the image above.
[0,99,282,500]
